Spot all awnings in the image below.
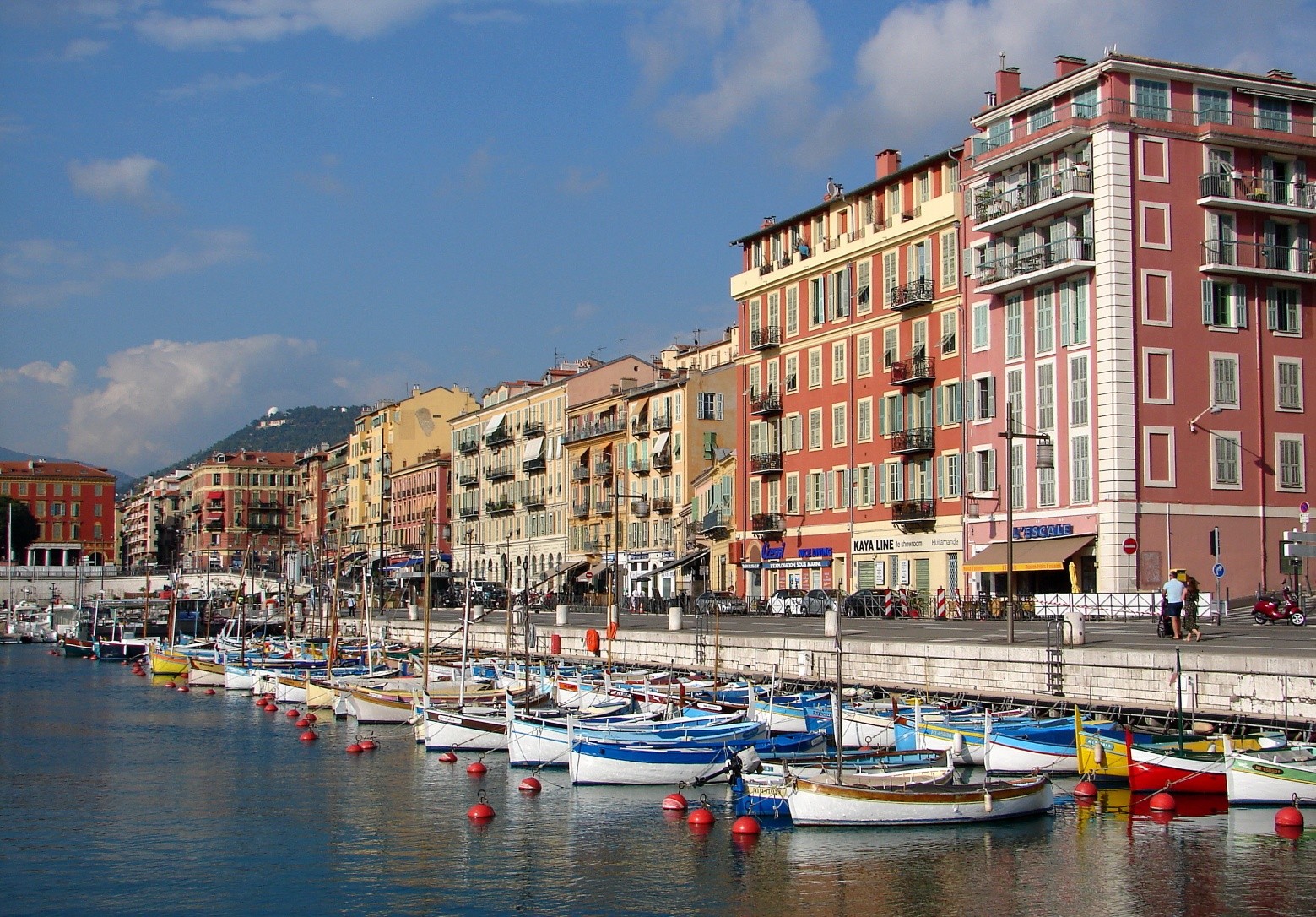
[576,562,615,582]
[544,562,587,576]
[302,555,439,569]
[639,547,712,580]
[963,535,1097,572]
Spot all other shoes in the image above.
[1196,633,1202,642]
[1183,639,1190,642]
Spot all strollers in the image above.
[1157,593,1183,641]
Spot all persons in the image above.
[729,586,734,596]
[631,587,638,596]
[347,594,356,618]
[1180,577,1202,642]
[1162,570,1186,639]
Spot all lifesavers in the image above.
[606,623,617,640]
[266,599,278,608]
[529,622,536,648]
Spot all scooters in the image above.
[1250,578,1306,627]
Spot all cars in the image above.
[800,588,850,618]
[765,589,808,617]
[695,590,748,616]
[843,588,902,617]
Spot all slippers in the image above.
[1171,636,1180,639]
[1178,635,1183,637]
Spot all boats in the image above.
[0,501,1316,835]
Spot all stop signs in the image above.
[1123,537,1137,554]
